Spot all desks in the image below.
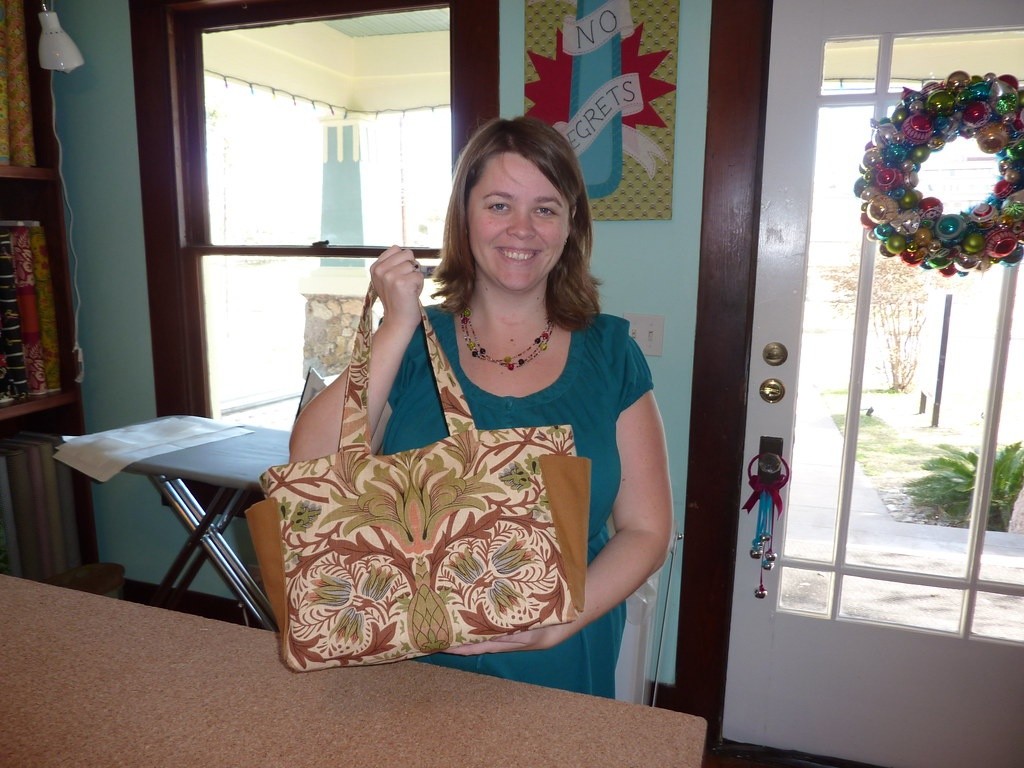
[0,575,711,768]
[57,422,290,631]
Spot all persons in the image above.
[290,117,671,700]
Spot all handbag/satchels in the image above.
[245,281,592,672]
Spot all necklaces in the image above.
[458,299,554,369]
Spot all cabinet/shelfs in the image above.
[0,0,98,584]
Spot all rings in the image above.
[409,258,419,271]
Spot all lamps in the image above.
[37,0,84,74]
